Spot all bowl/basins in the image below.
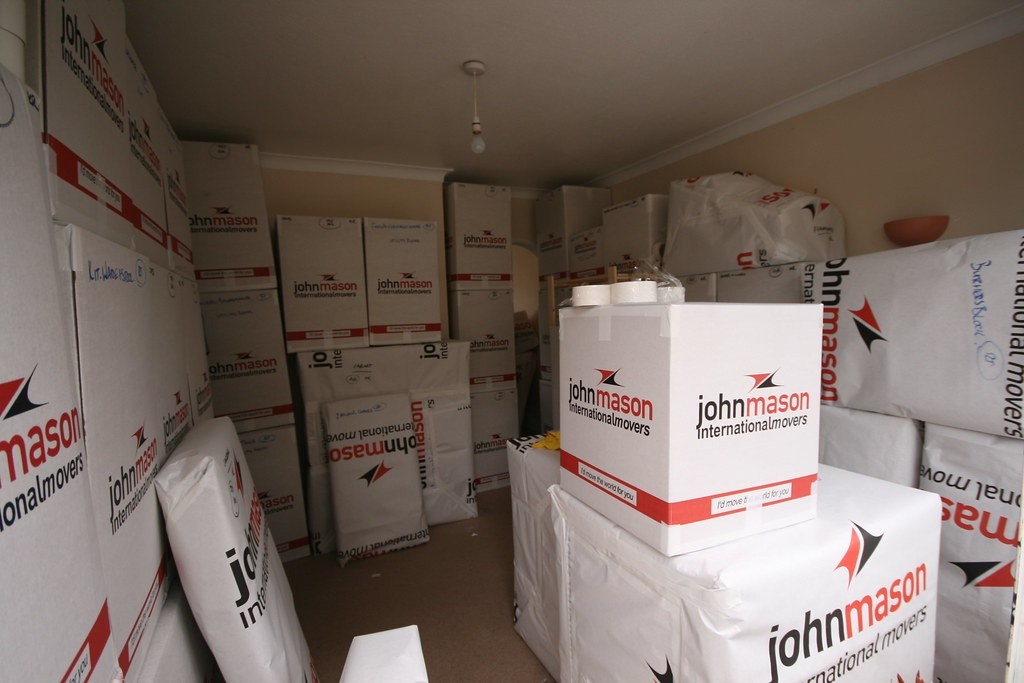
[884,215,950,247]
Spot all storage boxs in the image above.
[0,0,1024,683]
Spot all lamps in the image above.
[463,60,486,153]
